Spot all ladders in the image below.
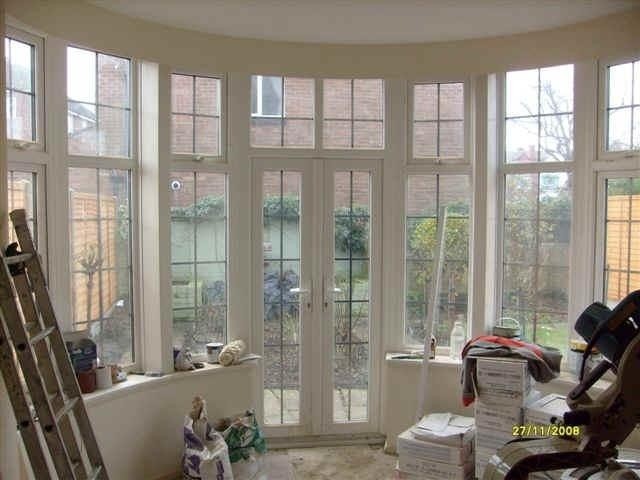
[0,209,108,480]
[0,243,86,480]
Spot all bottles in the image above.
[451,322,464,360]
[429,339,435,360]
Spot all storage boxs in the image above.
[398,454,474,480]
[394,468,475,480]
[474,446,499,478]
[474,391,541,434]
[397,424,472,466]
[410,418,473,448]
[476,358,531,409]
[474,425,524,450]
[524,393,572,439]
